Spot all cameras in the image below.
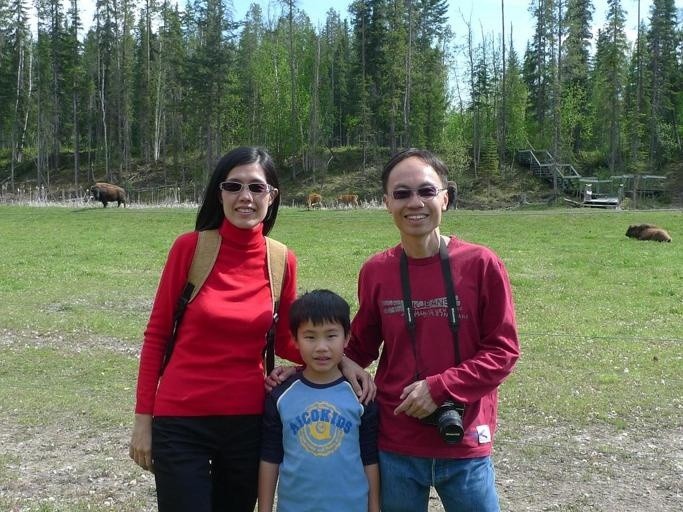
[420,399,465,444]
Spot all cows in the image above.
[89,181,128,208]
[337,195,358,209]
[625,222,672,243]
[304,191,322,212]
[445,180,458,211]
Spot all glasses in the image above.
[388,186,448,200]
[219,181,278,195]
